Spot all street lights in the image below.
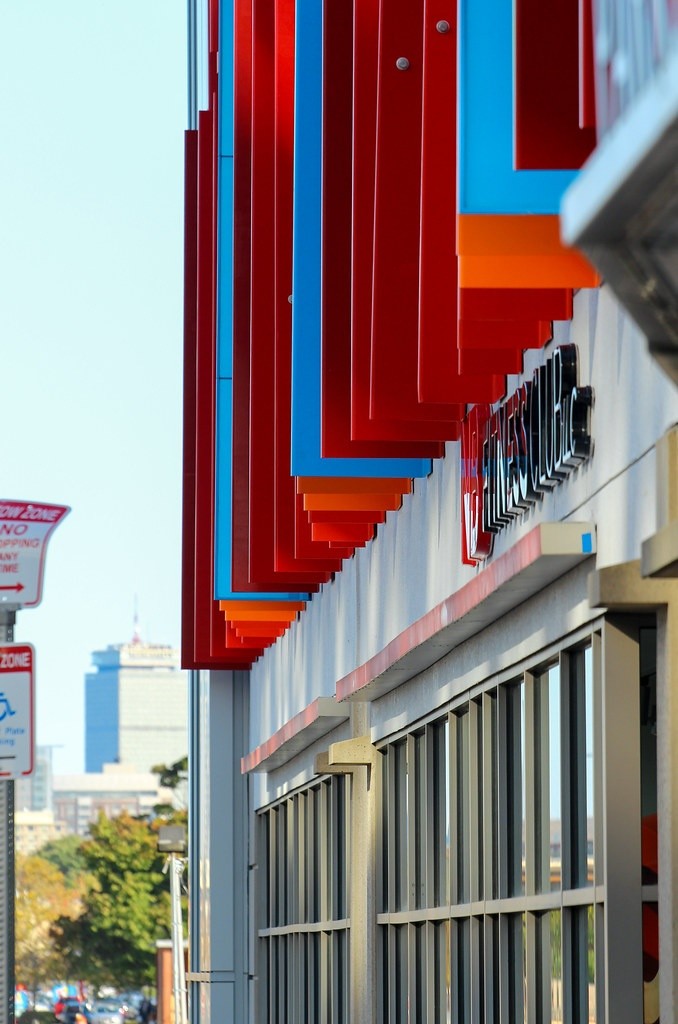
[156,826,187,1024]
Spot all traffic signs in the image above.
[0,499,71,608]
